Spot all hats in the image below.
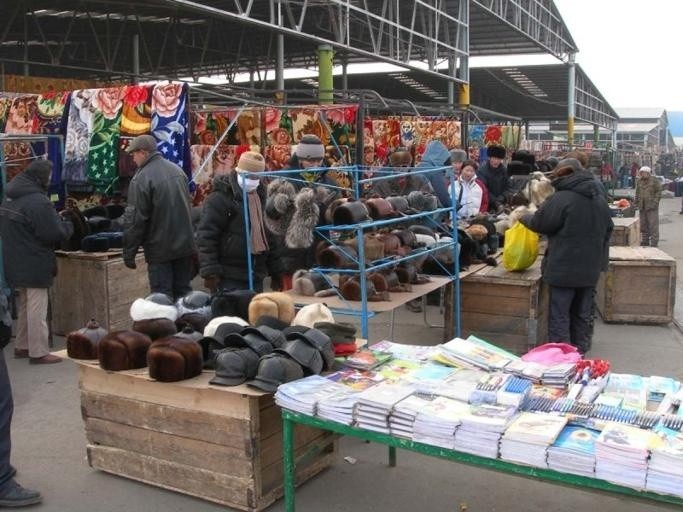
[124,134,157,156]
[209,349,257,387]
[388,150,413,167]
[97,329,153,372]
[245,353,304,393]
[486,145,506,159]
[508,149,586,178]
[638,165,652,174]
[57,202,124,251]
[321,229,420,271]
[292,263,429,301]
[131,287,356,355]
[66,316,110,361]
[235,150,265,177]
[144,335,204,383]
[328,188,442,225]
[448,149,467,162]
[294,134,325,159]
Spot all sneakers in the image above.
[1,477,48,509]
[14,346,31,359]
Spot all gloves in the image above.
[124,257,137,270]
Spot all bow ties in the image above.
[28,353,61,365]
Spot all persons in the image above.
[0,159,69,363]
[516,151,615,357]
[122,134,196,302]
[367,141,663,247]
[196,151,268,295]
[0,291,42,508]
[268,134,343,292]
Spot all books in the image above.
[275,333,683,500]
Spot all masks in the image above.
[237,174,260,192]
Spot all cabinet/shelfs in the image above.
[240,165,462,346]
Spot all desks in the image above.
[609,204,635,217]
[282,408,683,512]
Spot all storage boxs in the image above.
[594,246,677,324]
[77,364,341,512]
[610,217,640,246]
[50,250,151,336]
[445,255,550,357]
